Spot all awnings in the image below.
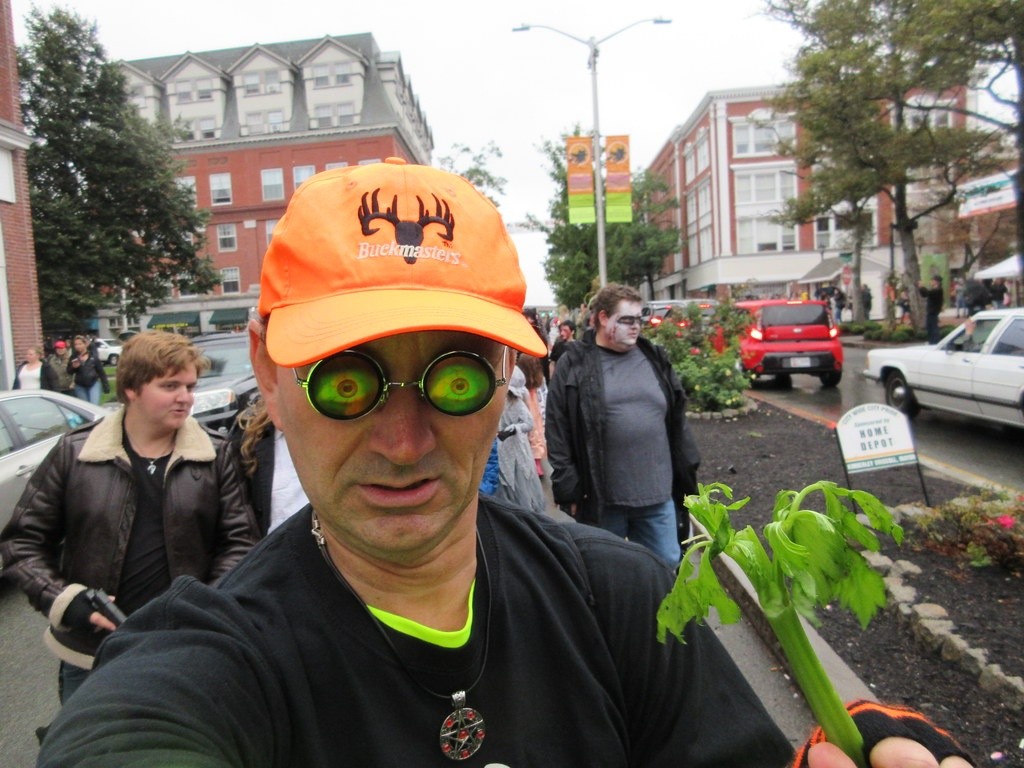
[209,308,248,324]
[147,311,200,328]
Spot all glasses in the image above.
[291,345,513,423]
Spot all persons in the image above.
[817,284,872,323]
[227,307,578,547]
[951,277,1012,319]
[0,330,260,705]
[12,334,110,406]
[38,157,979,768]
[918,275,944,344]
[801,288,809,300]
[546,280,699,578]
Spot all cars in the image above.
[187,331,263,437]
[863,307,1024,435]
[0,388,124,573]
[91,338,125,367]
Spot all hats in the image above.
[257,157,549,361]
[55,340,67,350]
[507,364,529,400]
[930,274,943,283]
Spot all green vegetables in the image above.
[655,480,907,768]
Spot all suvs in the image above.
[639,298,721,338]
[720,296,844,391]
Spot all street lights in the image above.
[512,16,675,290]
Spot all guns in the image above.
[92,588,127,627]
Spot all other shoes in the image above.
[536,459,545,478]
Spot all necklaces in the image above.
[310,513,493,761]
